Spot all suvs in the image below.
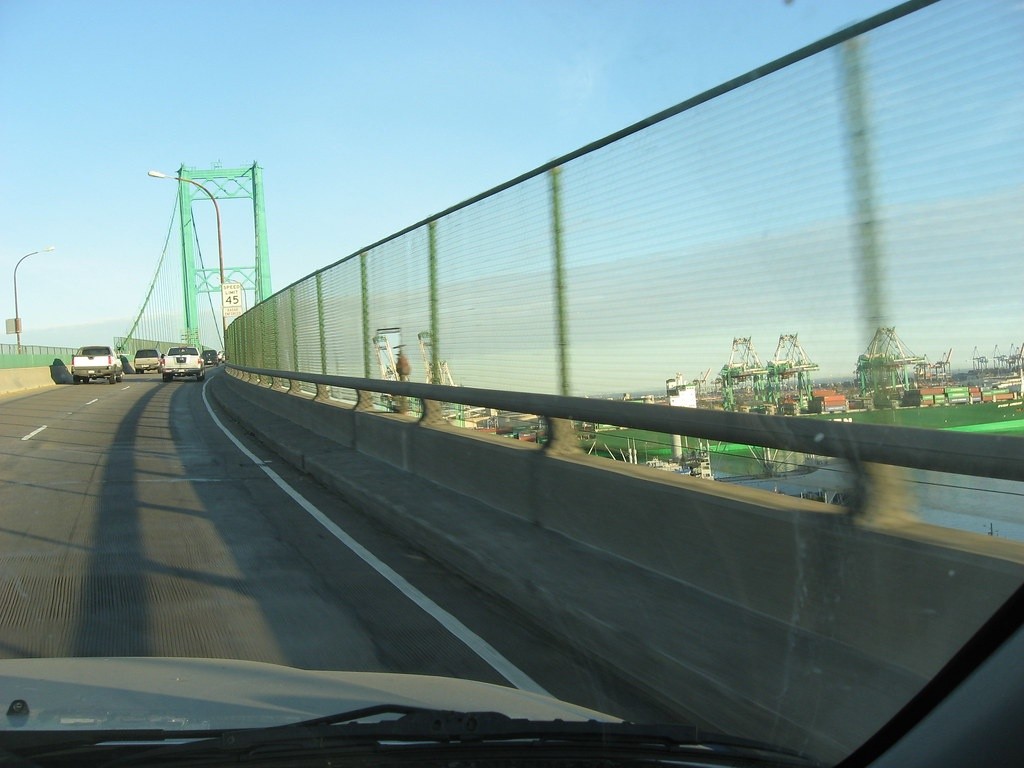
[201,349,218,366]
[161,346,207,382]
[134,347,164,374]
[70,345,125,385]
[217,350,226,363]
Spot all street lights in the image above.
[13,246,55,355]
[147,170,227,360]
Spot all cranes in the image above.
[695,325,1024,417]
